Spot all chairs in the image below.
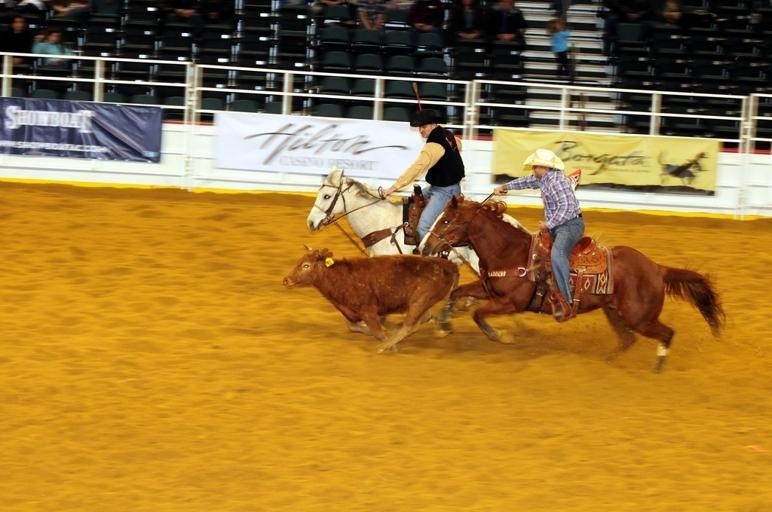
[597,0,767,147]
[0,2,528,127]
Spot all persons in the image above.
[380,108,466,245]
[0,0,237,99]
[494,148,587,318]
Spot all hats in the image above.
[409,109,447,127]
[523,148,565,171]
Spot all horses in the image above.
[306,168,531,276]
[413,191,727,375]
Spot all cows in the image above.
[282,242,460,355]
